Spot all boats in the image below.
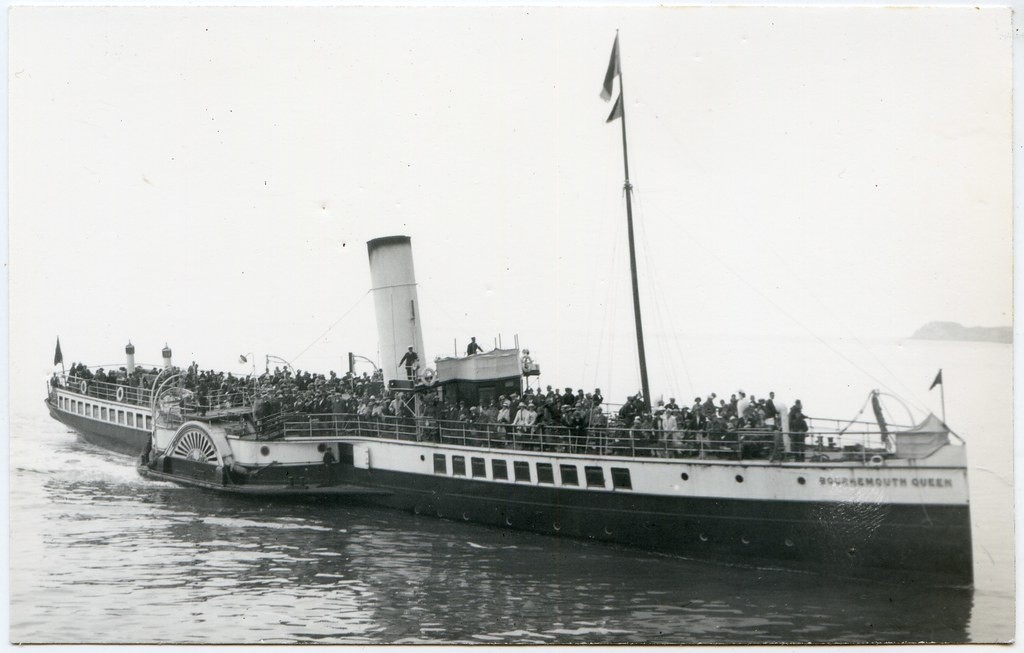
[36,232,974,575]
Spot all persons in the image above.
[47,337,810,460]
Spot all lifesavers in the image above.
[117,387,124,401]
[81,381,87,394]
[422,367,436,386]
[521,355,533,372]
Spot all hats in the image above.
[518,402,526,407]
[528,404,535,406]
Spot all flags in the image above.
[599,32,623,124]
[929,370,941,389]
[53,337,63,365]
